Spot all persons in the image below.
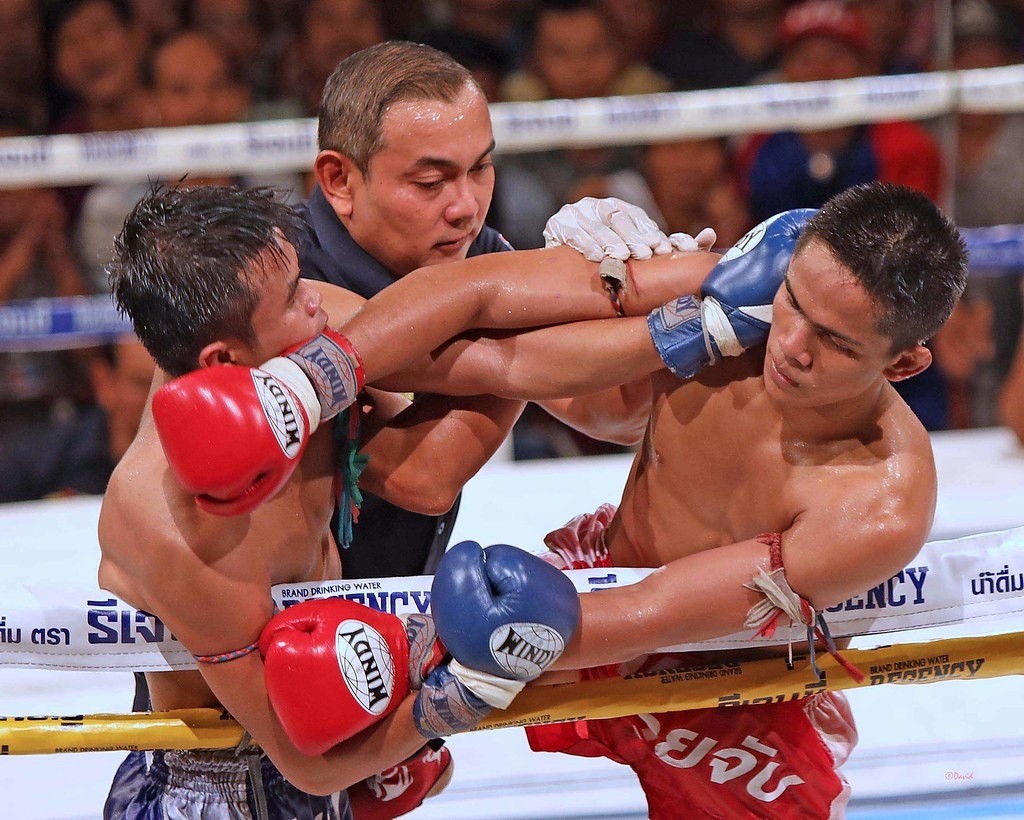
[271,42,716,579]
[98,175,821,820]
[151,179,968,820]
[0,0,1024,506]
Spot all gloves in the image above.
[414,541,583,743]
[259,600,448,756]
[648,208,821,380]
[543,197,717,263]
[152,326,364,516]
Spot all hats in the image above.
[783,1,878,50]
